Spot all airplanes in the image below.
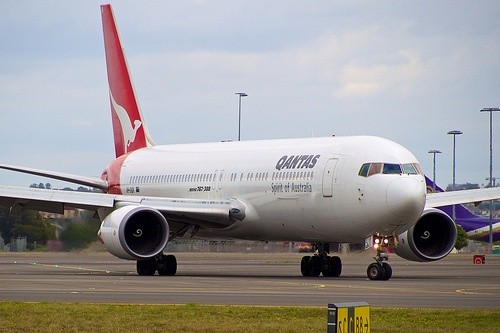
[0,4,500,282]
